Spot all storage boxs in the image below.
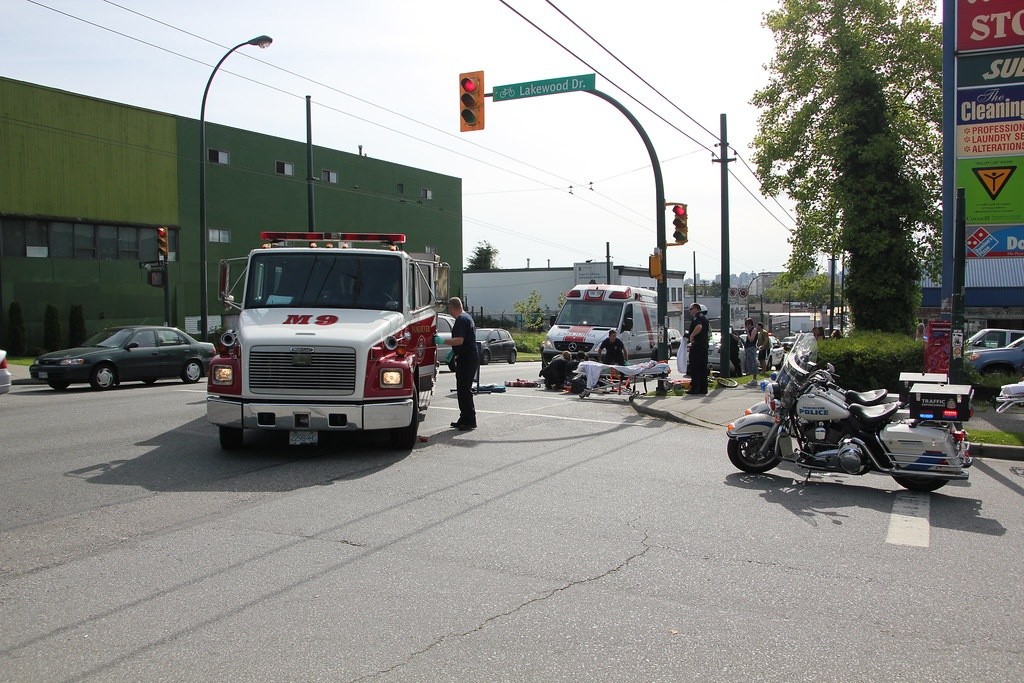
[911,383,974,422]
[898,371,947,405]
[882,425,962,474]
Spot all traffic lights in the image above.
[648,255,663,278]
[459,70,486,134]
[672,205,688,242]
[156,226,169,255]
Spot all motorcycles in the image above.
[724,331,977,493]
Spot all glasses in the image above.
[690,306,696,310]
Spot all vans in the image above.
[961,328,1024,355]
[540,283,658,365]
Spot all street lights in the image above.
[747,273,771,318]
[199,34,274,341]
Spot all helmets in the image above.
[700,304,707,312]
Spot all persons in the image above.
[729,324,742,377]
[597,330,632,393]
[576,351,585,362]
[917,323,925,337]
[540,351,571,390]
[755,322,769,374]
[683,303,713,394]
[812,327,825,340]
[434,297,481,431]
[831,329,843,339]
[744,318,759,386]
[584,353,589,361]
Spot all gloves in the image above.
[434,336,444,344]
[446,351,454,362]
[683,333,689,338]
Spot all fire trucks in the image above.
[205,230,454,450]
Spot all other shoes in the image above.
[685,384,708,394]
[747,379,757,386]
[451,418,477,430]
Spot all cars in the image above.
[436,312,458,372]
[666,327,682,359]
[964,336,1024,380]
[738,331,785,371]
[685,332,746,375]
[782,336,796,351]
[27,325,218,391]
[475,327,518,366]
